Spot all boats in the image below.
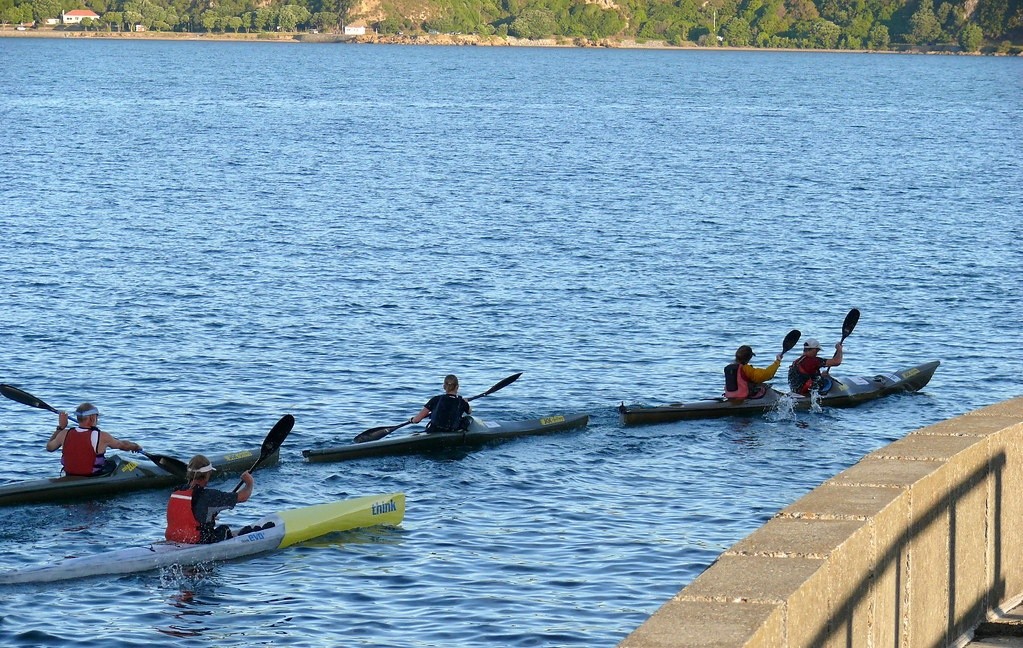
[0,446,279,509]
[622,359,940,425]
[0,489,405,580]
[302,410,591,459]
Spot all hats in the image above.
[738,346,756,357]
[187,463,217,473]
[804,338,825,351]
[75,408,105,417]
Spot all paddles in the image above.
[827,308,861,372]
[0,383,188,477]
[213,414,294,516]
[780,329,802,357]
[353,371,524,444]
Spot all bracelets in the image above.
[411,417,414,423]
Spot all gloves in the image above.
[409,417,419,424]
[777,353,783,360]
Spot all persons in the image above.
[788,338,843,396]
[46,403,142,477]
[165,455,254,544]
[409,374,474,435]
[724,345,783,399]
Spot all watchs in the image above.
[57,426,63,431]
